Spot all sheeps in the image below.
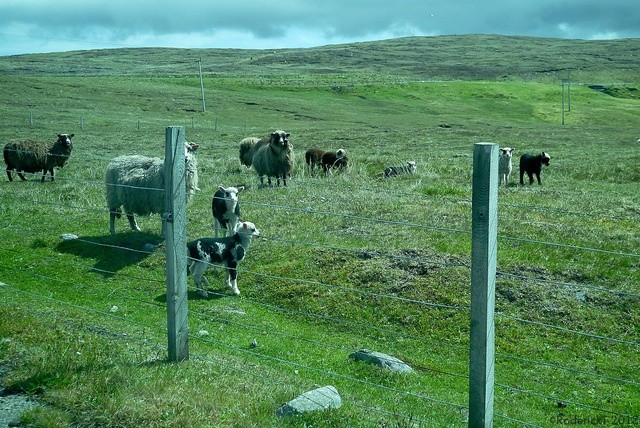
[383,160,417,179]
[104,139,202,236]
[186,221,262,298]
[322,148,349,176]
[498,147,516,186]
[239,136,261,167]
[252,130,296,187]
[3,133,75,182]
[519,151,552,186]
[305,148,325,177]
[212,186,245,238]
